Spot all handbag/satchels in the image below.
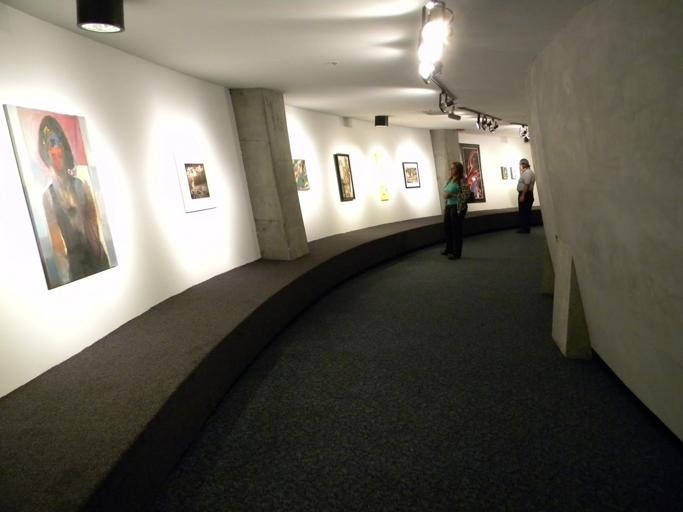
[458,178,475,204]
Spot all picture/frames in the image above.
[334,153,356,202]
[459,143,486,203]
[402,162,420,188]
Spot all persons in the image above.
[36,116,110,285]
[516,158,535,234]
[439,162,472,260]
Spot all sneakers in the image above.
[440,248,450,255]
[447,252,462,260]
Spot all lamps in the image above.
[417,0,461,120]
[375,116,388,126]
[76,0,124,33]
[511,123,529,142]
[458,107,501,132]
